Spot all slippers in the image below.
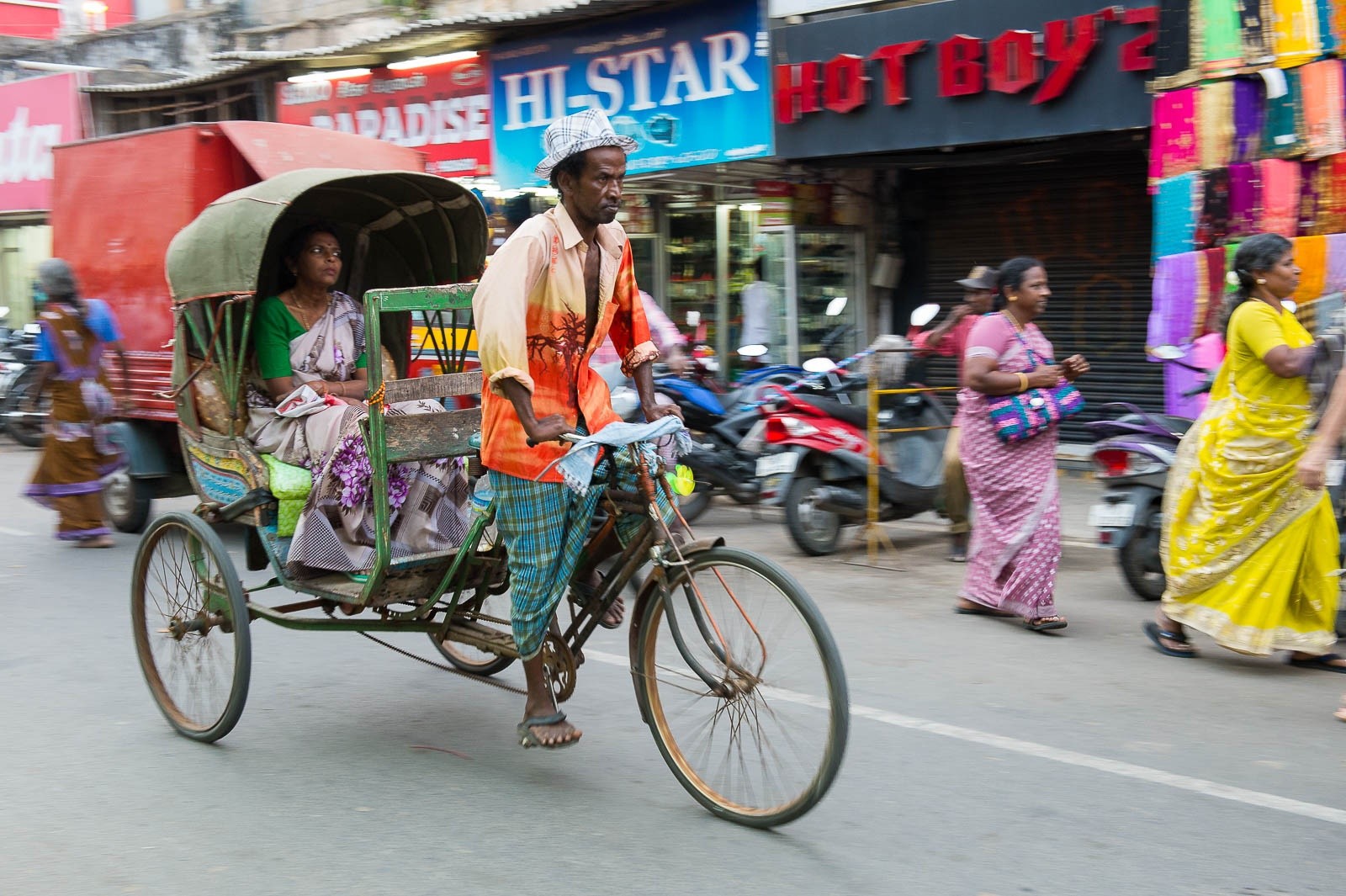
[517,710,579,750]
[1286,652,1346,674]
[1143,619,1197,658]
[568,570,624,630]
[343,570,368,583]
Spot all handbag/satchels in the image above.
[984,312,1089,446]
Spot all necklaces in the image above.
[1002,309,1023,334]
[289,290,314,331]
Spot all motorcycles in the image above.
[51,117,434,542]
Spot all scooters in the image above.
[652,295,952,557]
[1,305,56,450]
[1080,344,1216,603]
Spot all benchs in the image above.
[192,346,396,538]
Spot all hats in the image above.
[954,265,1002,290]
[533,107,639,180]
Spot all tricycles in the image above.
[127,167,852,830]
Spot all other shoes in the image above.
[945,538,967,563]
[952,599,1019,617]
[74,537,115,548]
[1024,615,1067,631]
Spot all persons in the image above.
[909,263,1000,569]
[1296,343,1346,720]
[953,256,1092,634]
[243,220,471,584]
[471,107,686,750]
[1144,229,1329,660]
[19,257,116,549]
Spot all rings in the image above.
[1082,363,1086,367]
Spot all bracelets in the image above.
[318,380,329,397]
[1014,372,1029,393]
[334,380,347,397]
